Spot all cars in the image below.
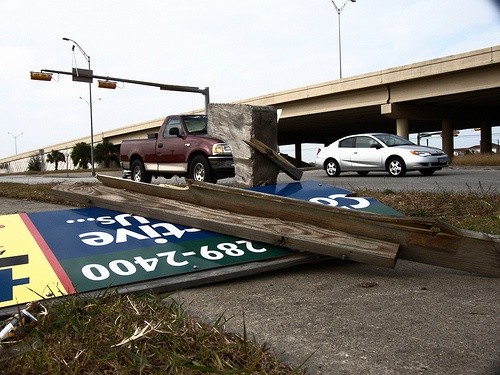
[316,132,449,178]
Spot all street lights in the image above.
[62,37,96,178]
[332,0,358,81]
[8,132,24,155]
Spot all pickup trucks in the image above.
[118,114,236,185]
